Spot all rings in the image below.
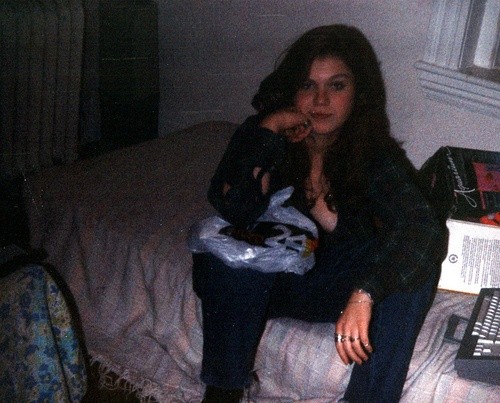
[304,119,311,127]
[335,334,360,343]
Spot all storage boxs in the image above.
[417,145,500,296]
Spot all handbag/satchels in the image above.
[184,185,319,275]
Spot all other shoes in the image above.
[201,385,244,403]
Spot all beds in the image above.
[24,121,500,403]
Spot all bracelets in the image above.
[346,289,374,306]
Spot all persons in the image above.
[192,25,451,403]
